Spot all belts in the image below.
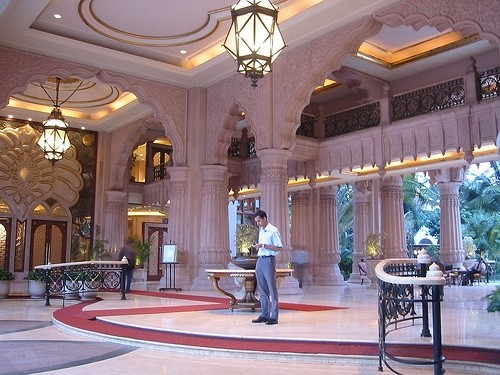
[258,255,274,258]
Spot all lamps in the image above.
[36,77,83,166]
[220,0,288,89]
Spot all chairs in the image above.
[443,265,480,288]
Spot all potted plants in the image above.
[129,154,138,182]
[24,269,102,301]
[132,236,156,282]
[0,269,18,298]
[462,236,477,269]
[228,223,258,297]
[363,231,386,289]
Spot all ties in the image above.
[476,263,480,269]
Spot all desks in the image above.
[451,270,466,285]
[206,269,295,312]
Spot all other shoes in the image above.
[252,316,269,323]
[126,290,130,293]
[266,318,278,325]
[469,283,473,286]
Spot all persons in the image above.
[251,210,284,326]
[118,238,136,293]
[461,257,486,286]
